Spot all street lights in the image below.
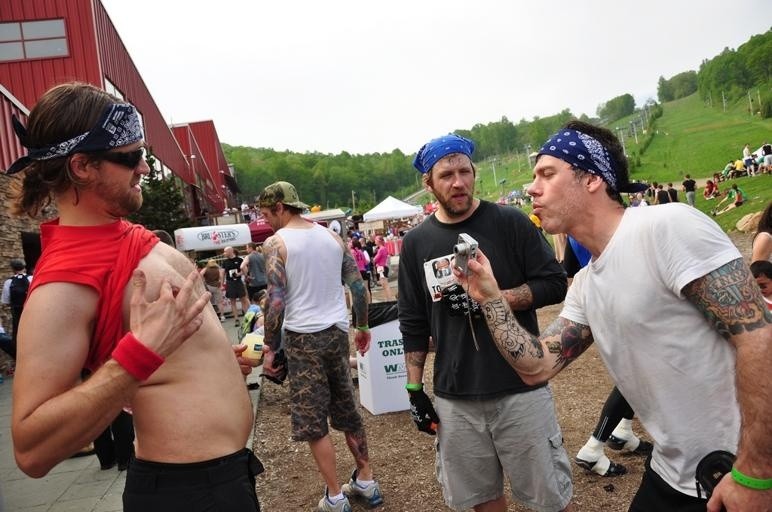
[491,157,497,189]
[500,178,506,206]
[615,100,655,162]
[351,189,357,212]
[524,142,532,169]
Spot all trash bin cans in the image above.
[352,300,411,416]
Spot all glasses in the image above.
[96,149,143,170]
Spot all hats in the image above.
[257,180,310,210]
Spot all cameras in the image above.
[452,232,478,276]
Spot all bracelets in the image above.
[730,464,771,494]
[356,324,370,331]
[402,382,424,392]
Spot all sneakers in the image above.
[341,469,383,506]
[574,448,627,478]
[316,485,352,512]
[605,426,654,457]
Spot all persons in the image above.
[260,182,388,512]
[452,121,771,511]
[398,134,575,511]
[11,80,254,511]
[80,367,137,474]
[576,383,653,478]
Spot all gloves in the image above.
[404,381,441,436]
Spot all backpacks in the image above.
[8,274,30,312]
